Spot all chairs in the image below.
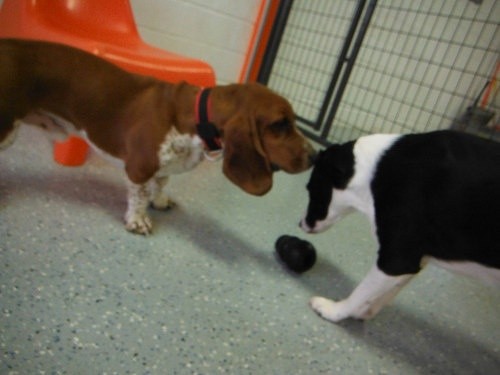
[0,0,215,167]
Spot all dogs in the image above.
[297,128,500,325]
[0,38,317,237]
